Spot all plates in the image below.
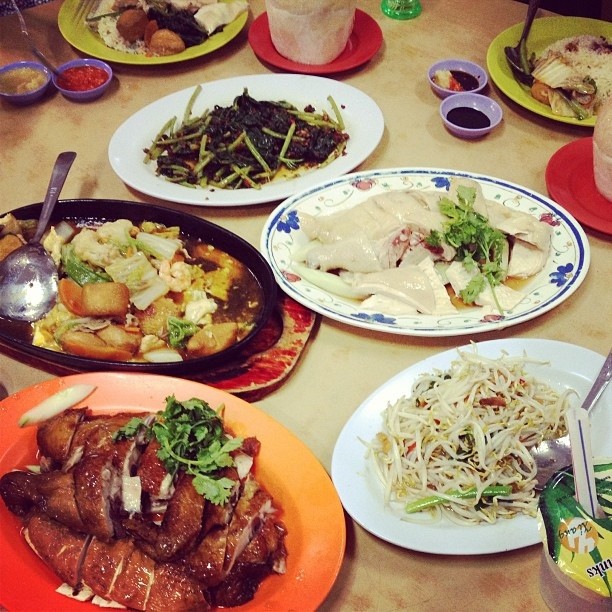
[487,17,611,127]
[1,199,275,376]
[261,166,591,338]
[57,1,248,65]
[248,7,382,75]
[545,136,612,236]
[1,371,346,608]
[109,74,384,208]
[330,336,612,555]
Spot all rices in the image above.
[541,35,612,112]
[93,1,151,56]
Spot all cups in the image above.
[536,461,611,607]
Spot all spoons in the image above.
[521,348,611,491]
[1,151,76,321]
[504,1,540,80]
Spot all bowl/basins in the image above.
[428,58,488,98]
[1,61,52,105]
[440,94,503,139]
[53,59,113,102]
